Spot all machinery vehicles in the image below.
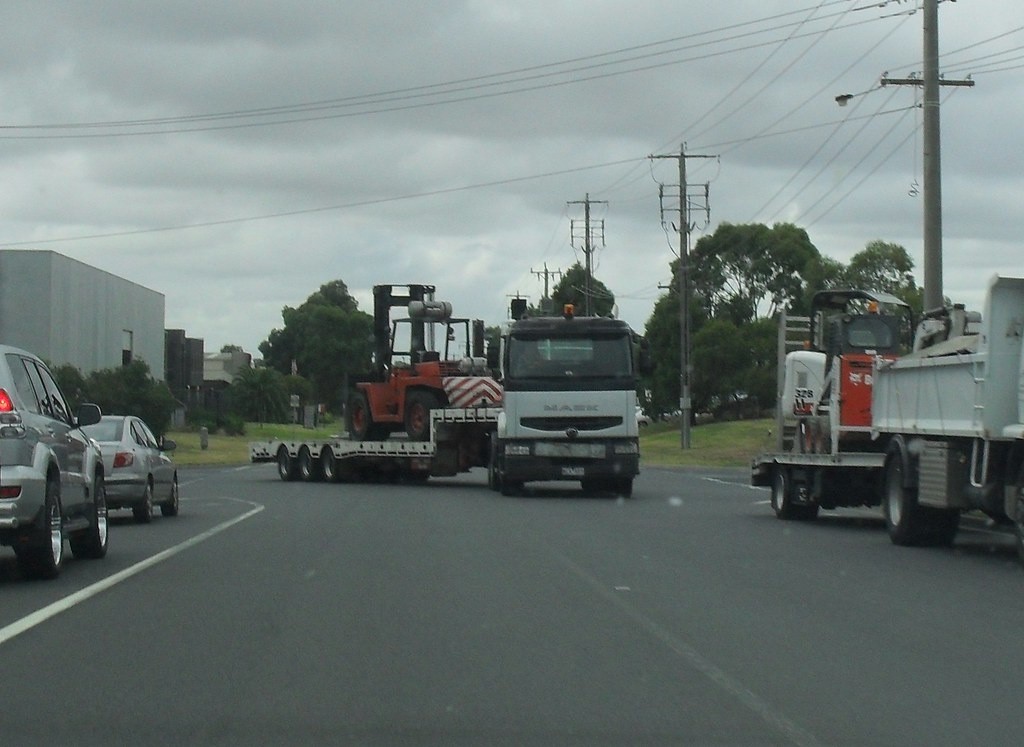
[343,283,504,440]
[780,289,913,450]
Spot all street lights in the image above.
[834,83,945,314]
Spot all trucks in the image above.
[870,274,1024,544]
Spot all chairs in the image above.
[419,351,440,364]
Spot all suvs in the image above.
[0,345,108,577]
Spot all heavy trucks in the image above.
[247,305,643,501]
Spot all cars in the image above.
[74,415,178,521]
[636,407,653,426]
